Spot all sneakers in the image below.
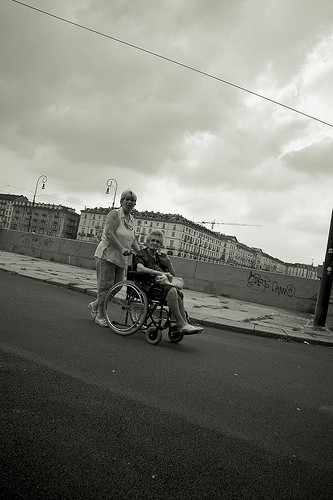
[88,302,98,319]
[95,317,108,327]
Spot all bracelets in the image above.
[155,270,158,275]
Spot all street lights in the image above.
[106,179,118,209]
[28,175,47,230]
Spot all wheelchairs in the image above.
[102,251,184,345]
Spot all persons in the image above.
[135,229,204,333]
[88,190,140,327]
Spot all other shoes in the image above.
[180,324,204,331]
